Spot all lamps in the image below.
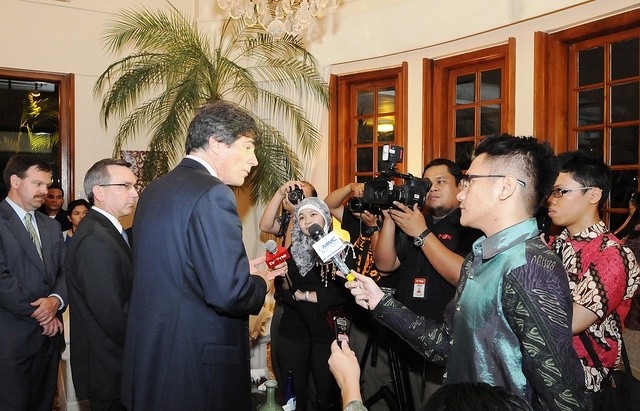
[215,1,347,37]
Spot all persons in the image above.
[332,133,598,410]
[372,156,484,409]
[323,180,385,289]
[61,160,138,410]
[121,101,285,410]
[541,148,639,400]
[60,193,90,242]
[325,336,542,411]
[261,179,319,407]
[0,152,68,410]
[267,196,357,410]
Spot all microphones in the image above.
[332,313,351,351]
[263,239,290,278]
[308,224,366,301]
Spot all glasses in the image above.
[458,173,526,190]
[93,182,138,191]
[550,185,602,197]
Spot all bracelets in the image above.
[305,291,310,302]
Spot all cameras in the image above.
[285,182,306,208]
[348,191,380,219]
[363,145,431,211]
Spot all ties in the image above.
[121,226,133,251]
[23,212,44,267]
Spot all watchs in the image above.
[39,182,70,222]
[413,237,423,248]
